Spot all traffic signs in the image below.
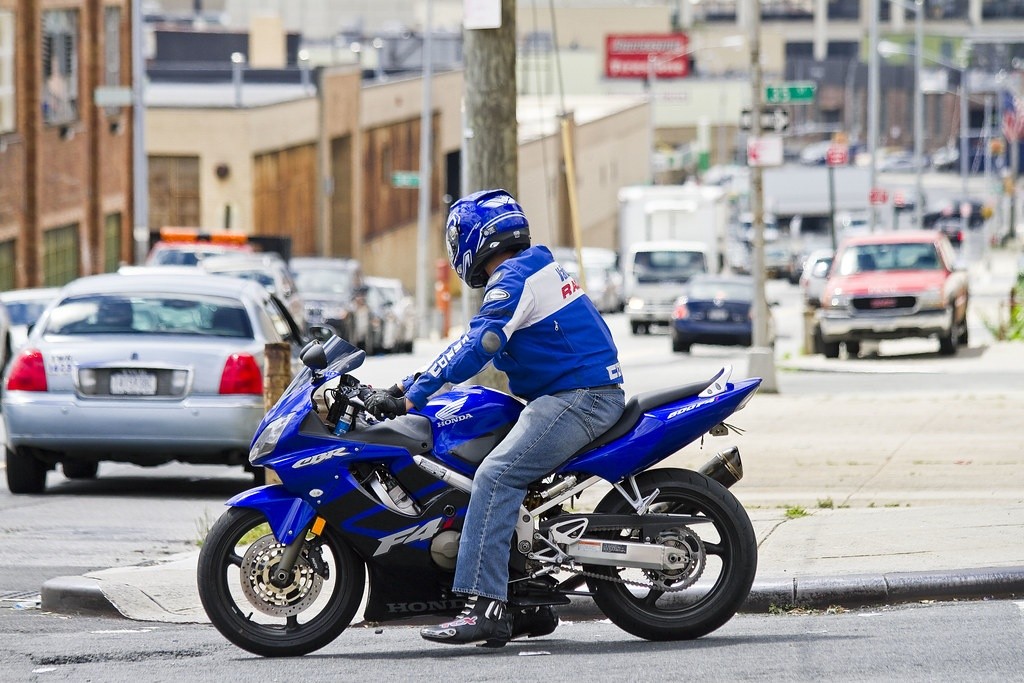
[740,108,790,134]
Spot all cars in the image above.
[666,274,779,352]
[543,244,624,316]
[735,208,834,309]
[0,267,334,496]
[0,228,416,388]
[817,229,970,359]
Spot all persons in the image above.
[365,188,626,646]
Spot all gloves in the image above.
[359,383,404,401]
[364,393,407,420]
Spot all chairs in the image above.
[211,307,260,340]
[94,297,134,332]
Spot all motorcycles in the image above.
[192,322,764,660]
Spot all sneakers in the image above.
[420,594,513,646]
[509,603,558,638]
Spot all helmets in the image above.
[445,188,530,288]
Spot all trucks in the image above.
[618,183,721,338]
